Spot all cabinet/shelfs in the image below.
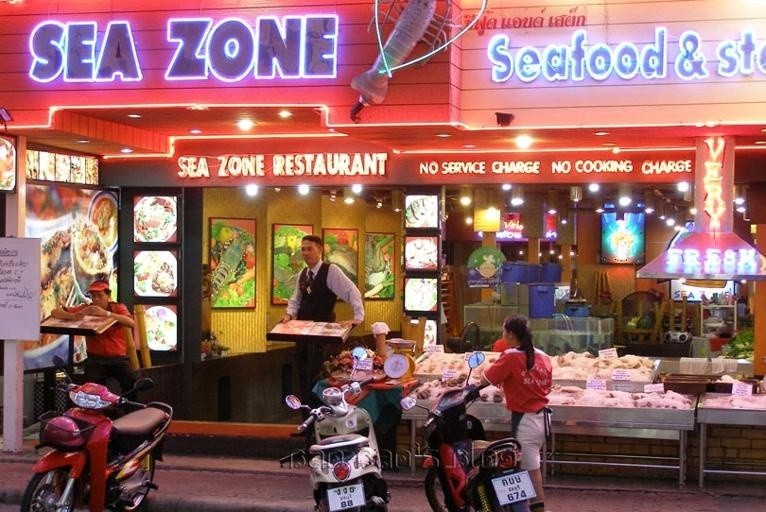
[701,301,736,337]
[697,392,766,488]
[402,396,698,486]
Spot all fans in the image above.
[447,322,480,352]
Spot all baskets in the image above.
[38,410,96,452]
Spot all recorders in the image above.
[664,329,693,344]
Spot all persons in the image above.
[277,235,366,421]
[51,282,139,403]
[480,316,552,512]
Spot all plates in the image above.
[134,250,177,297]
[0,138,16,191]
[405,279,438,311]
[405,240,437,269]
[143,306,178,351]
[133,196,177,242]
[87,190,119,255]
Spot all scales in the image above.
[384,338,415,384]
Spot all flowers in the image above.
[324,346,387,403]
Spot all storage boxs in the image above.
[501,262,543,284]
[540,262,561,281]
[501,284,529,306]
[529,284,558,318]
[565,304,588,316]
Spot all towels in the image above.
[712,359,736,373]
[680,357,707,374]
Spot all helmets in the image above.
[42,415,86,447]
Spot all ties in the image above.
[308,271,313,280]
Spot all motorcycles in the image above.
[286,347,393,510]
[20,357,174,511]
[399,353,539,512]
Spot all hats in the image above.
[87,280,109,292]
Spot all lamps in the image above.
[735,185,746,204]
[736,204,747,212]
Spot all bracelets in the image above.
[286,314,292,321]
[106,310,111,317]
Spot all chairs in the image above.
[689,337,709,358]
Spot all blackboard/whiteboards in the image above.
[0,238,42,341]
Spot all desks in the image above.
[625,342,689,355]
[312,377,419,445]
